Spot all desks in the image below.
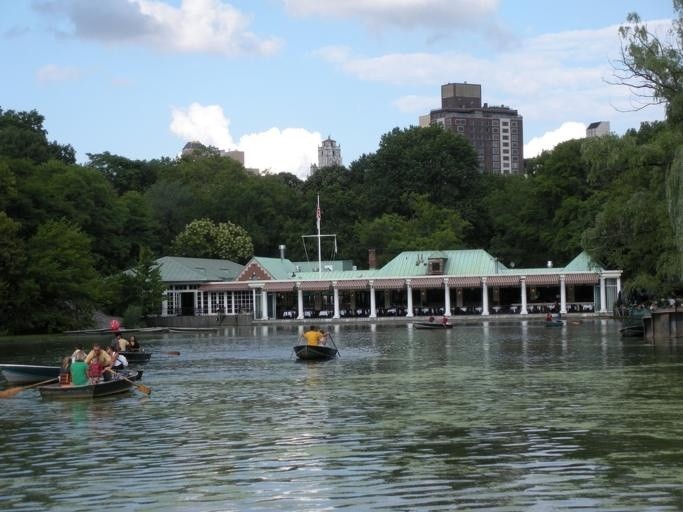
[283,304,595,319]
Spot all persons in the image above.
[556,313,561,320]
[543,301,559,313]
[638,303,656,312]
[442,316,448,325]
[319,330,326,344]
[302,325,329,345]
[61,333,140,384]
[429,315,435,324]
[546,311,552,321]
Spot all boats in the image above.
[0,365,63,385]
[413,321,452,330]
[538,317,566,328]
[292,346,337,362]
[166,326,220,333]
[113,351,151,365]
[39,371,139,400]
[618,325,644,337]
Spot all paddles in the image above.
[102,366,150,396]
[0,376,59,399]
[138,350,181,355]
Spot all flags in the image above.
[317,207,321,229]
